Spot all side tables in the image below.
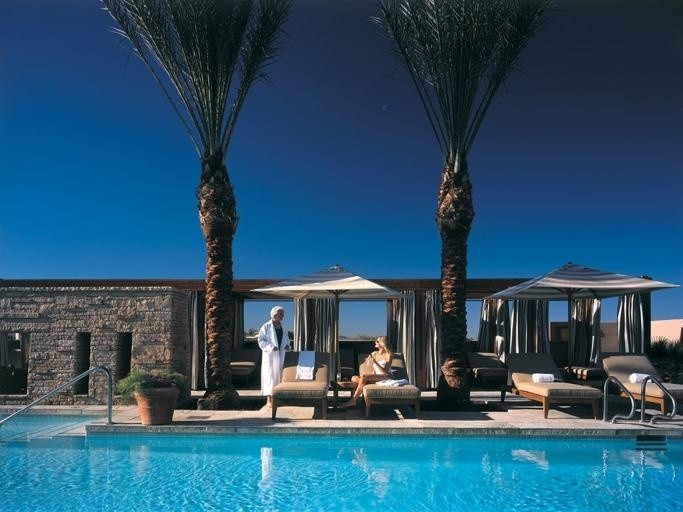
[331,381,357,412]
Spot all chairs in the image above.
[271,352,330,419]
[358,353,421,419]
[230,360,256,387]
[341,349,355,374]
[469,352,683,419]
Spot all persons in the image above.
[340,336,393,408]
[257,306,292,408]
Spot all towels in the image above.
[376,379,408,387]
[296,351,315,380]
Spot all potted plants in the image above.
[113,369,190,425]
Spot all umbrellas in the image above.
[249,264,408,408]
[483,261,681,363]
